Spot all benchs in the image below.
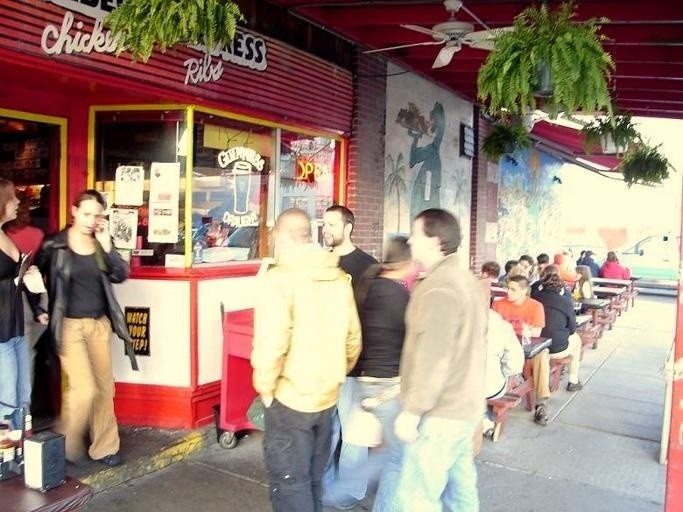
[488,277,643,443]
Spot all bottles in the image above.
[522,328,531,345]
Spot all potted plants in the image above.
[580,105,641,158]
[476,0,620,130]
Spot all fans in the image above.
[361,0,516,69]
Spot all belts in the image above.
[486,382,506,400]
[63,311,105,318]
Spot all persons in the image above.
[253,210,361,512]
[480,250,631,440]
[1,179,38,439]
[12,187,44,266]
[315,206,485,512]
[26,191,131,468]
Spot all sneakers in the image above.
[535,404,549,425]
[568,381,583,390]
[103,455,120,466]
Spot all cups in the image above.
[233,161,252,215]
[573,303,581,316]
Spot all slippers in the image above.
[484,420,501,442]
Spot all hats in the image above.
[586,251,596,256]
